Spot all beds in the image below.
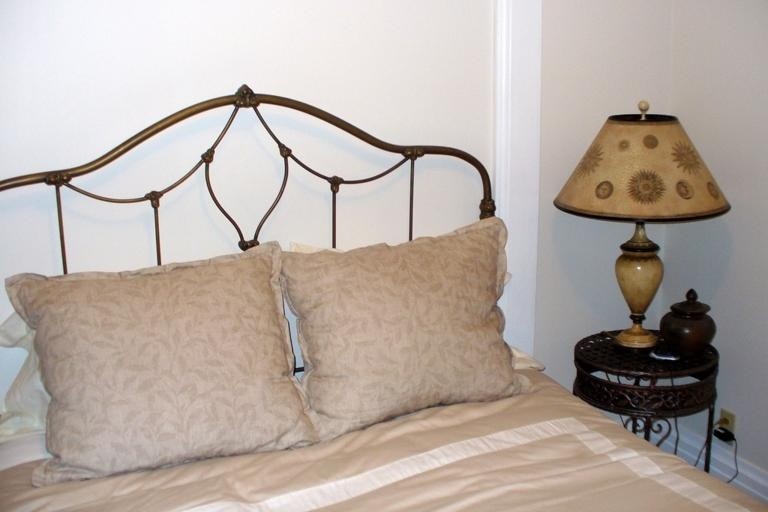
[0,82,768,512]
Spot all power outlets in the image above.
[719,408,736,438]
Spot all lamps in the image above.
[553,100,731,348]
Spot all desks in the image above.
[572,328,719,473]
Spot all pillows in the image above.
[0,308,52,474]
[279,217,536,441]
[5,241,317,487]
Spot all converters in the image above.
[713,425,733,444]
